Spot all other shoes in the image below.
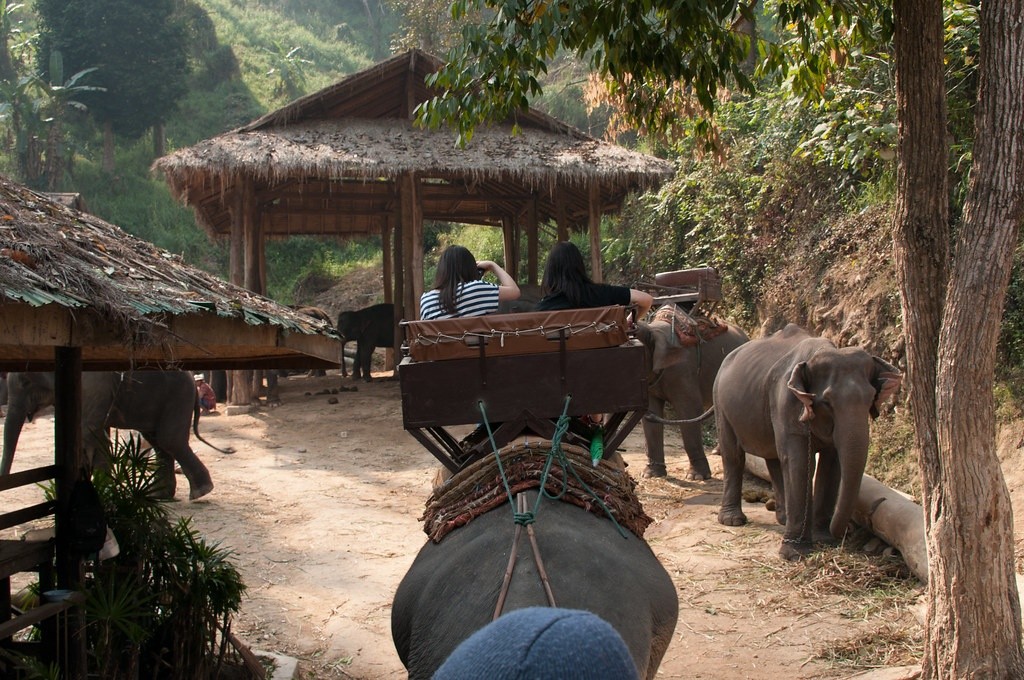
[200,410,210,415]
[588,413,606,426]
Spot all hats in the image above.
[194,374,204,381]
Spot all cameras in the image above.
[478,268,484,276]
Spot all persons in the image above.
[534,241,653,426]
[420,245,521,321]
[193,372,216,416]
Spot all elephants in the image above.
[211,306,332,406]
[391,488,678,680]
[0,371,236,500]
[644,322,902,563]
[632,304,750,480]
[336,304,406,382]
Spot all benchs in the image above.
[632,265,721,317]
[398,304,648,474]
